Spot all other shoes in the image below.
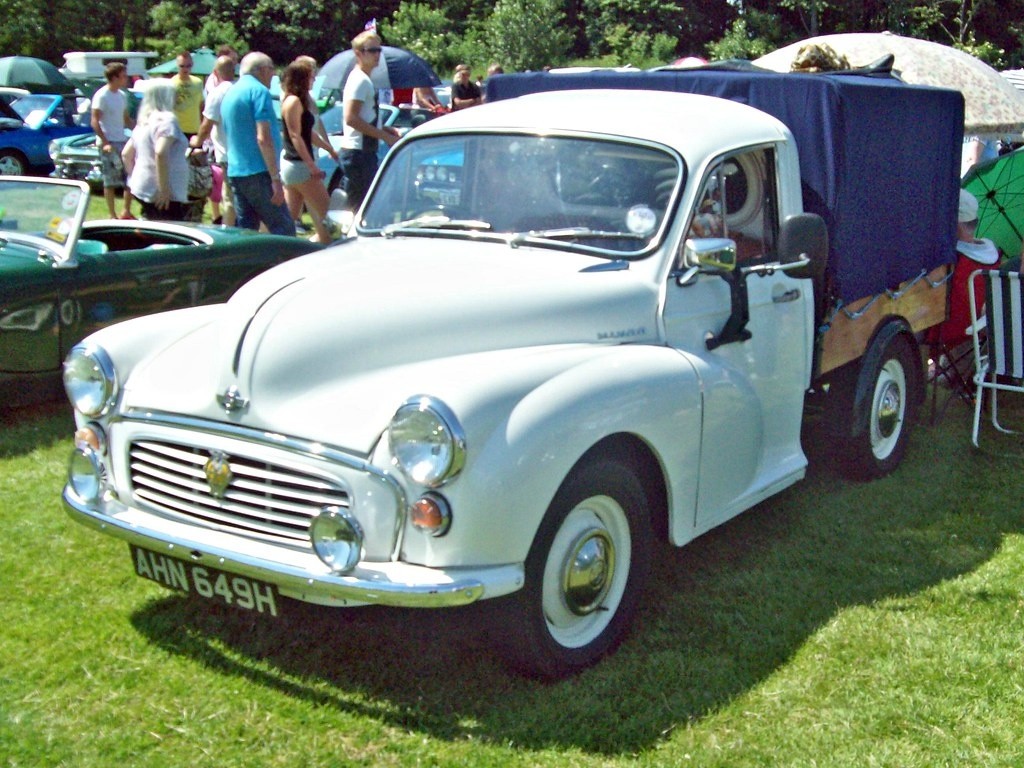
[121,213,137,220]
[295,222,308,235]
[214,216,224,226]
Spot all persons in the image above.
[483,150,633,251]
[411,87,442,118]
[122,45,296,236]
[544,66,551,72]
[451,65,504,112]
[999,137,1024,156]
[650,165,755,259]
[342,32,402,220]
[280,57,338,245]
[914,189,1000,344]
[91,63,137,220]
[523,69,533,73]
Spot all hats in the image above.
[957,188,979,224]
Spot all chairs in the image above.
[966,269,1024,461]
[920,247,1003,429]
[76,239,109,254]
[513,214,620,251]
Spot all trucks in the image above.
[57,58,966,682]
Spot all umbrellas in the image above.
[961,147,1024,262]
[316,48,442,88]
[71,77,138,117]
[675,57,706,67]
[0,56,75,93]
[751,31,1024,142]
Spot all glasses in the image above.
[179,63,193,69]
[360,48,382,54]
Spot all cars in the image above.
[1,173,321,412]
[1,84,467,218]
